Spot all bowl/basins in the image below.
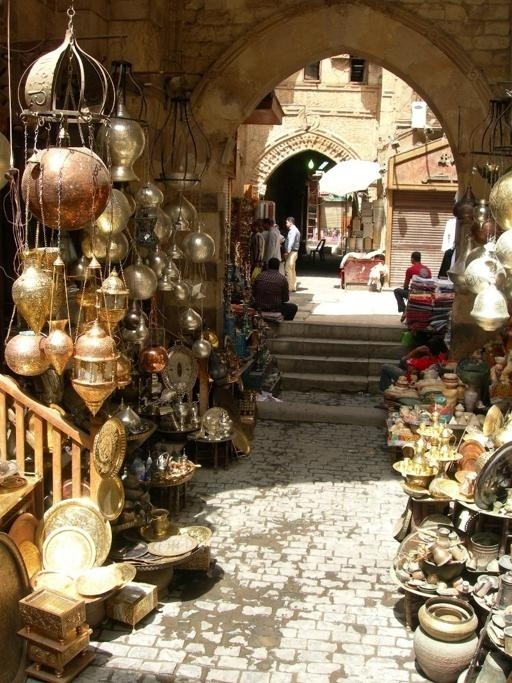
[394,369,459,406]
[418,596,479,642]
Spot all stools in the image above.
[143,430,236,514]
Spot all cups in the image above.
[438,527,449,542]
[428,542,452,567]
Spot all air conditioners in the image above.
[411,101,443,129]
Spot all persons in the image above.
[249,220,265,275]
[250,258,298,320]
[262,218,283,270]
[393,251,432,322]
[283,216,301,290]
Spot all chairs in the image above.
[311,239,326,262]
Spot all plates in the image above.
[28,417,198,598]
[397,563,474,599]
